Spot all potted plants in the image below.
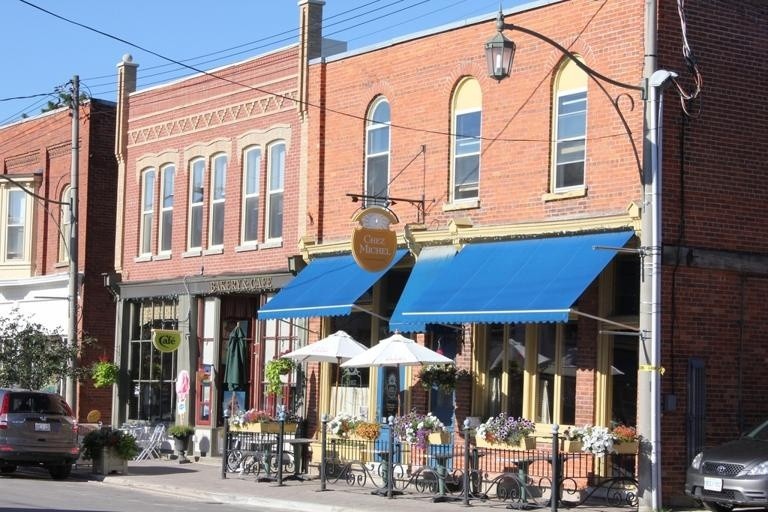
[80,426,140,476]
[263,357,295,396]
[168,425,195,453]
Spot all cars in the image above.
[0,387,82,480]
[683,418,768,512]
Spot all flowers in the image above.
[322,411,383,446]
[225,407,275,425]
[419,364,467,396]
[390,410,638,455]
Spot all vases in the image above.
[228,420,297,433]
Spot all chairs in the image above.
[136,424,167,463]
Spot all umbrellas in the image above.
[223,321,250,418]
[339,328,457,418]
[543,347,625,379]
[486,340,549,372]
[282,329,370,417]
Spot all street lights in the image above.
[484,2,657,512]
